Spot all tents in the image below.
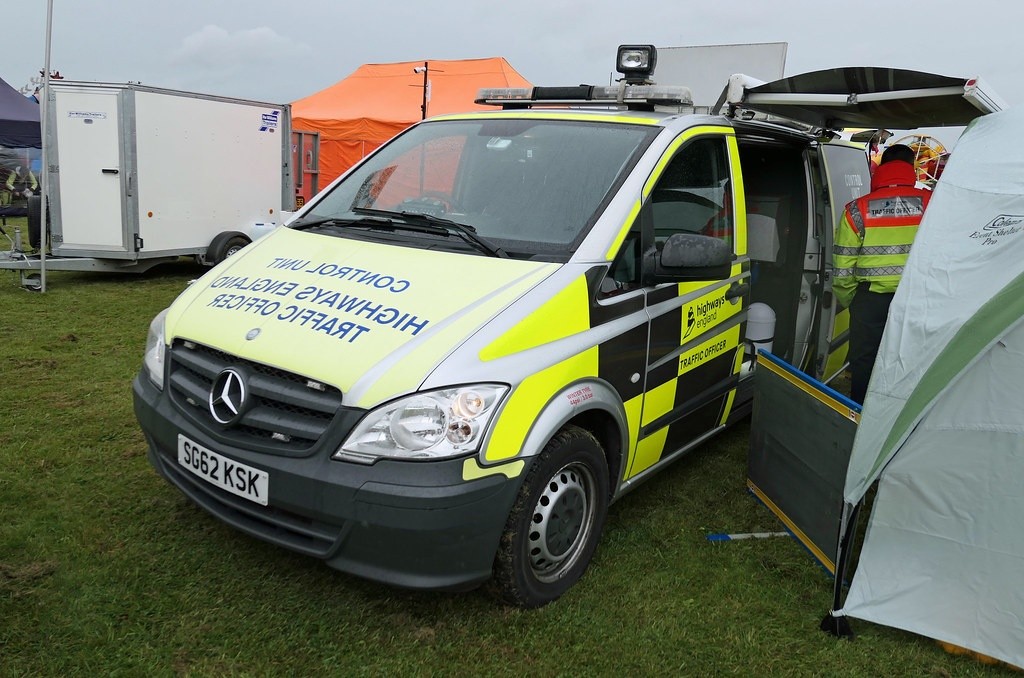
[820,103,1024,672]
[286,57,572,215]
[0,76,43,149]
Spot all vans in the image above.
[124,37,1012,609]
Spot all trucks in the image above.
[0,76,318,269]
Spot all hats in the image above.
[16,165,31,181]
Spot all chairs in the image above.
[741,162,791,266]
[646,163,707,245]
[475,160,542,224]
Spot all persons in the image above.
[831,144,932,406]
[5,164,38,201]
[701,177,733,253]
